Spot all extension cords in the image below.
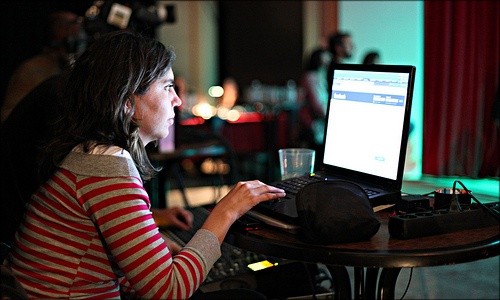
[388,202,500,241]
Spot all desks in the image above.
[197,182,500,299]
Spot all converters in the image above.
[397,192,430,213]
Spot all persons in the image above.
[0,28,287,300]
[323,31,354,98]
[0,7,195,247]
[296,47,334,166]
[362,50,414,136]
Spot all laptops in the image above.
[254,63,416,226]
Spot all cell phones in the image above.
[243,256,280,275]
